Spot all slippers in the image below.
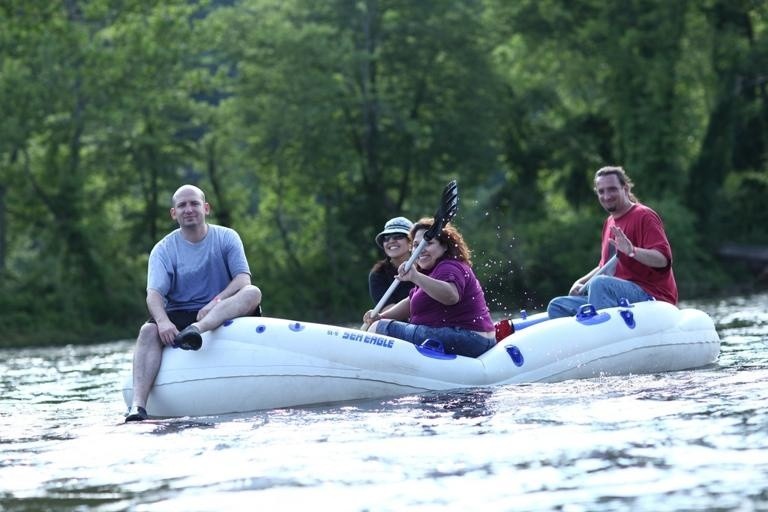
[171,325,203,351]
[125,406,148,423]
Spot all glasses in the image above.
[380,234,407,242]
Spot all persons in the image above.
[548,165,678,318]
[363,217,499,358]
[125,183,262,422]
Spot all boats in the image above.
[120,298,722,418]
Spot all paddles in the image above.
[360,181,458,332]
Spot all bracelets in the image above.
[628,245,637,258]
[214,295,221,304]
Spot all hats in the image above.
[375,216,413,248]
